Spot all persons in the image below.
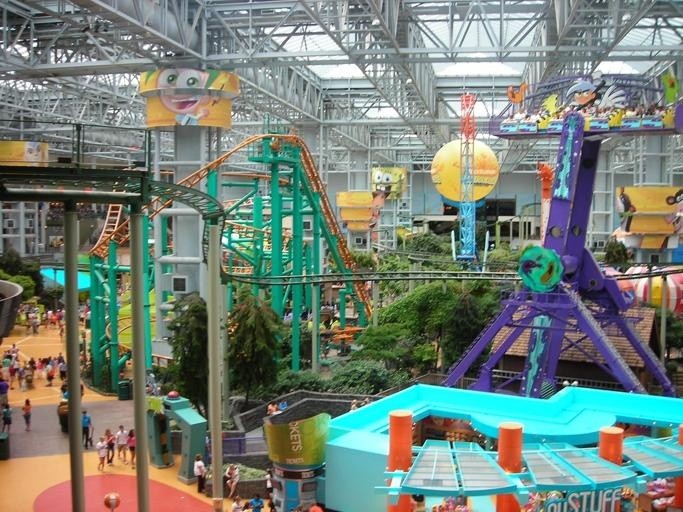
[193,453,207,494]
[231,493,277,512]
[18,304,92,343]
[1,343,94,450]
[505,102,666,119]
[95,424,137,471]
[225,463,240,498]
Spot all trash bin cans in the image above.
[118,380,130,400]
[0,432,9,460]
